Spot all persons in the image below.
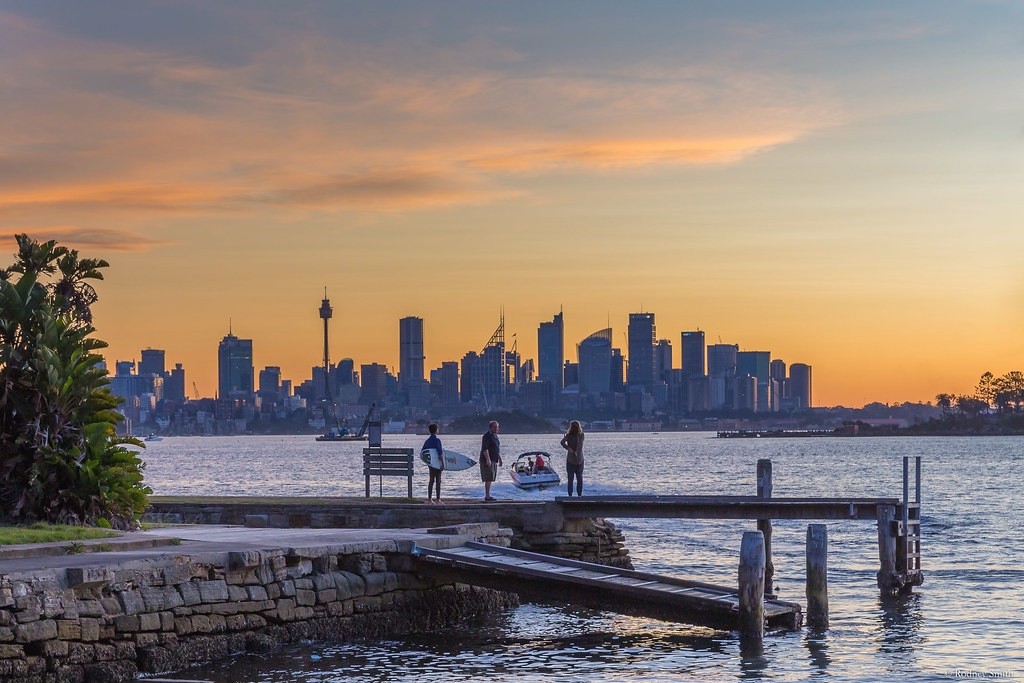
[420,424,447,506]
[533,455,544,475]
[527,457,534,474]
[560,420,585,497]
[480,421,503,502]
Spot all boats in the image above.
[510,452,560,488]
[145,433,163,441]
[315,404,374,441]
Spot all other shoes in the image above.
[428,500,436,505]
[484,497,498,502]
[436,500,444,505]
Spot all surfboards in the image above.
[420,448,477,471]
